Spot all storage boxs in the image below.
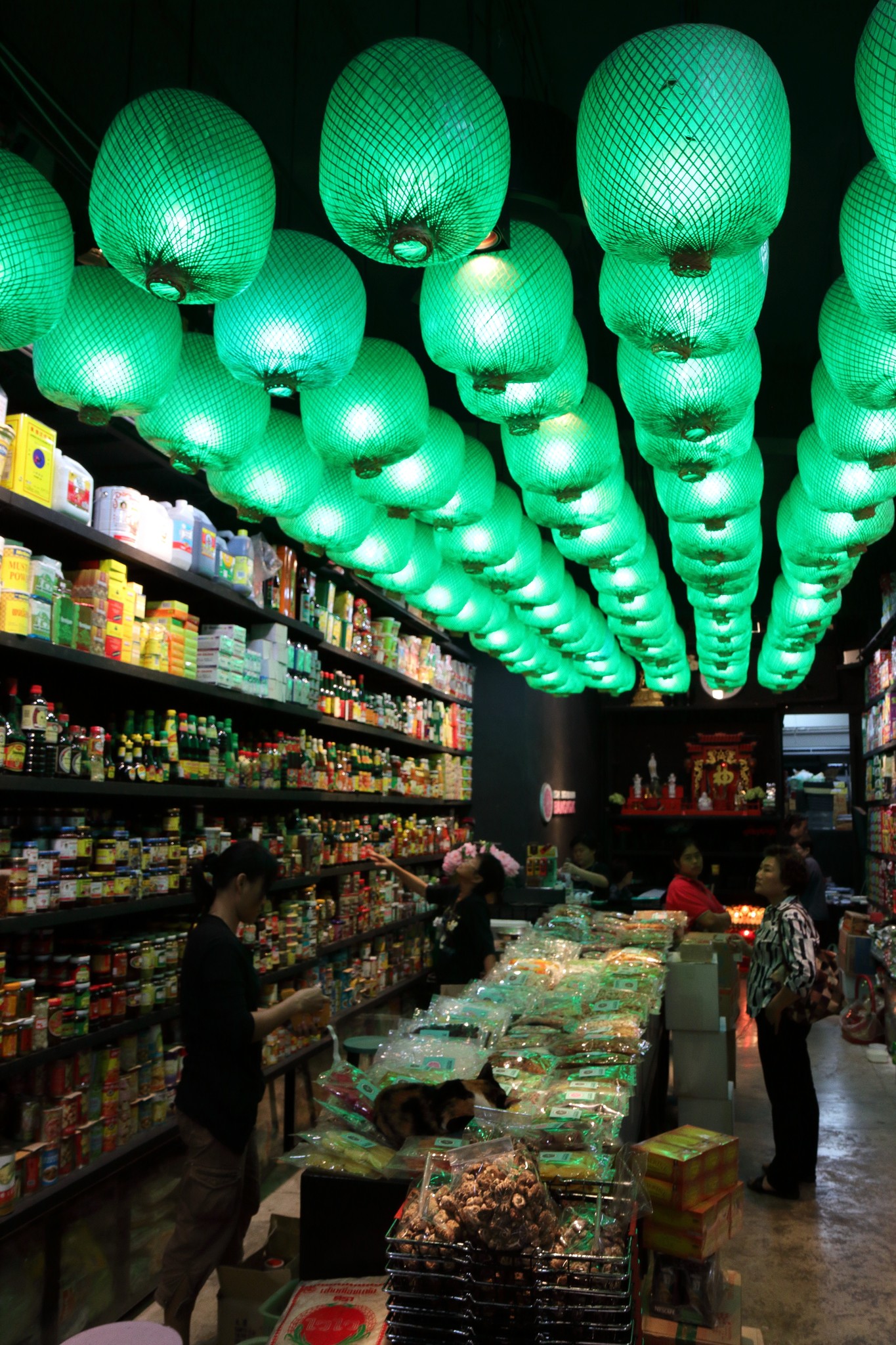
[839,911,872,1007]
[628,932,743,1345]
[216,1214,299,1345]
[803,781,853,832]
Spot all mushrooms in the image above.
[392,1150,625,1306]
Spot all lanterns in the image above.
[855,0,896,183]
[85,86,274,304]
[840,157,896,332]
[316,35,512,269]
[124,272,896,699]
[33,265,183,426]
[213,228,367,397]
[577,24,793,277]
[0,149,75,352]
[599,238,769,363]
[420,222,574,394]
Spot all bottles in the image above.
[264,572,280,610]
[334,670,353,721]
[374,692,402,734]
[349,815,374,862]
[288,809,324,866]
[351,744,382,795]
[318,670,333,715]
[104,709,177,783]
[402,695,435,742]
[418,817,456,856]
[374,816,398,859]
[158,499,253,596]
[397,816,417,859]
[0,677,104,784]
[322,818,348,865]
[253,742,280,789]
[351,675,378,726]
[435,760,444,799]
[300,729,327,791]
[326,742,352,793]
[276,731,301,789]
[297,566,310,623]
[381,747,416,797]
[420,758,431,796]
[177,713,253,787]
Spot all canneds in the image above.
[0,806,430,1208]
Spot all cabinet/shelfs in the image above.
[0,347,470,1345]
[853,612,896,1063]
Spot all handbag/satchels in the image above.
[778,906,846,1025]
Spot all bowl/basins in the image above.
[496,926,526,940]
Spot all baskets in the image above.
[842,974,883,1045]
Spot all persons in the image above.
[561,833,609,911]
[727,846,823,1201]
[663,834,732,934]
[777,814,827,949]
[154,839,329,1345]
[366,848,522,1110]
[612,822,635,916]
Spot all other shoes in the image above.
[762,1162,816,1183]
[747,1176,799,1199]
